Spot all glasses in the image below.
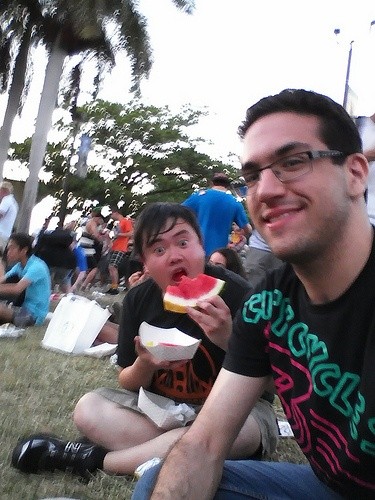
[228,151,350,197]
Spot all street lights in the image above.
[333,20,375,110]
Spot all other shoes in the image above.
[106,287,119,294]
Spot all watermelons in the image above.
[163,273,226,313]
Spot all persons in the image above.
[131,89,375,500]
[180,173,252,263]
[0,233,51,328]
[10,201,280,477]
[59,206,289,293]
[1,180,18,261]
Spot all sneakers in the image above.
[10,432,102,474]
